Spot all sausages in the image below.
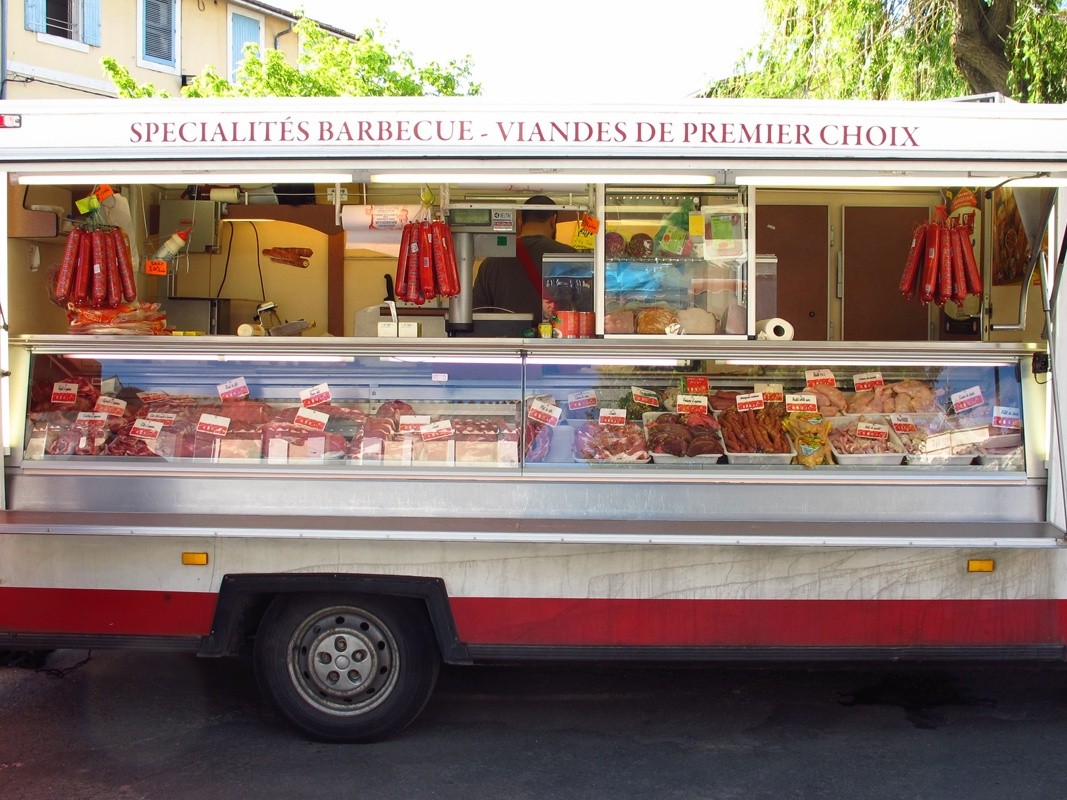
[55,223,137,310]
[394,216,462,309]
[709,390,794,453]
[263,246,313,269]
[899,222,983,309]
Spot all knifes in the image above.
[383,273,398,323]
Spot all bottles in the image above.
[151,226,191,263]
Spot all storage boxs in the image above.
[398,321,422,337]
[377,321,397,338]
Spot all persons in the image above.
[465,194,586,338]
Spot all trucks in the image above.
[0,98,1067,745]
